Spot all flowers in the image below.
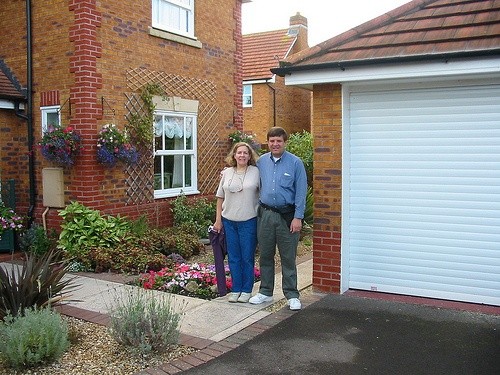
[0,181,30,239]
[96,124,137,166]
[31,123,86,168]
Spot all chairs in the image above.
[0,179,18,254]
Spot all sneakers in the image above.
[249,293,272,304]
[286,298,301,310]
[237,292,252,302]
[228,292,241,302]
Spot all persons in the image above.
[212,142,261,303]
[219,127,307,310]
